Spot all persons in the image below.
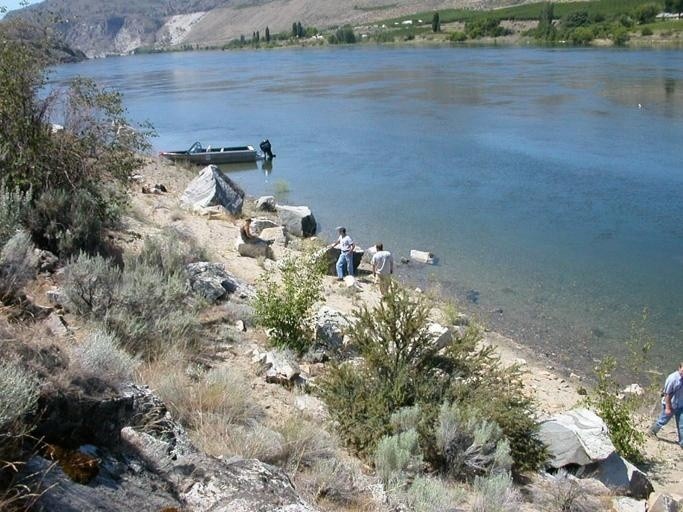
[644,361,683,449]
[240,218,275,247]
[141,183,175,195]
[370,243,395,295]
[327,226,356,281]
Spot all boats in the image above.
[159,141,257,165]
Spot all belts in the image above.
[342,249,350,253]
